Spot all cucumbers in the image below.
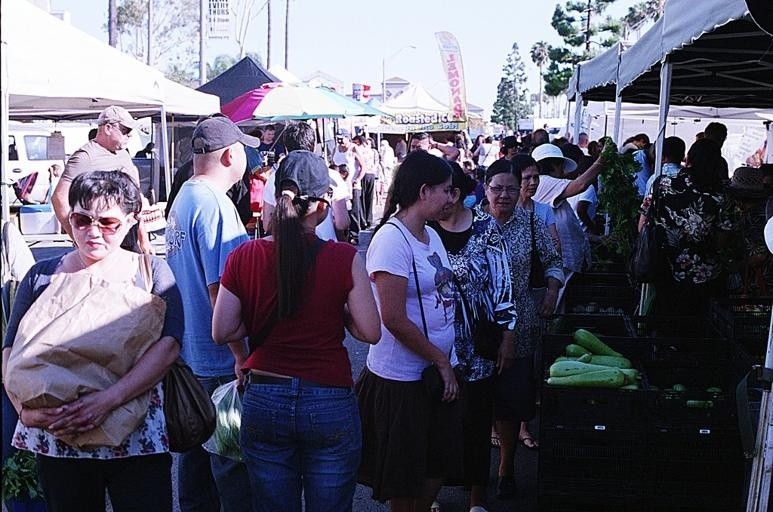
[212,407,243,460]
[547,329,641,390]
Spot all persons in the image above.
[1,104,772,512]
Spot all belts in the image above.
[248,373,291,386]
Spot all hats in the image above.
[98,104,139,131]
[532,143,578,173]
[275,149,338,206]
[720,166,769,198]
[190,117,261,155]
[500,135,523,155]
[334,128,349,139]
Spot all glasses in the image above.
[488,185,522,196]
[68,210,122,235]
[326,186,334,199]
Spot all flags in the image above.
[433,30,468,122]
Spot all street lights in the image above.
[381,44,416,101]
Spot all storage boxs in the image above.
[19,204,61,235]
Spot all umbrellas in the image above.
[219,82,377,128]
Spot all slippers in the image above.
[490,436,501,449]
[519,436,538,450]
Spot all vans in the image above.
[7,121,142,209]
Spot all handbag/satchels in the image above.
[528,211,546,292]
[628,175,672,285]
[1,221,21,346]
[162,353,218,453]
[421,364,461,414]
[471,319,502,361]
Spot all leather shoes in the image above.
[495,473,517,499]
